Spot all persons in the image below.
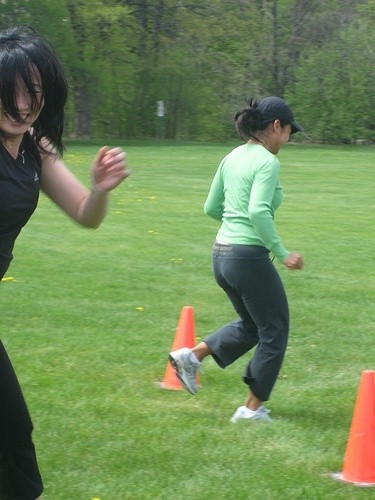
[0,24,133,500]
[167,96,304,424]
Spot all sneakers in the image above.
[167,346,203,398]
[230,403,273,425]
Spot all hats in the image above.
[252,95,302,134]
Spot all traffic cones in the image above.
[324,369,375,488]
[154,305,203,390]
